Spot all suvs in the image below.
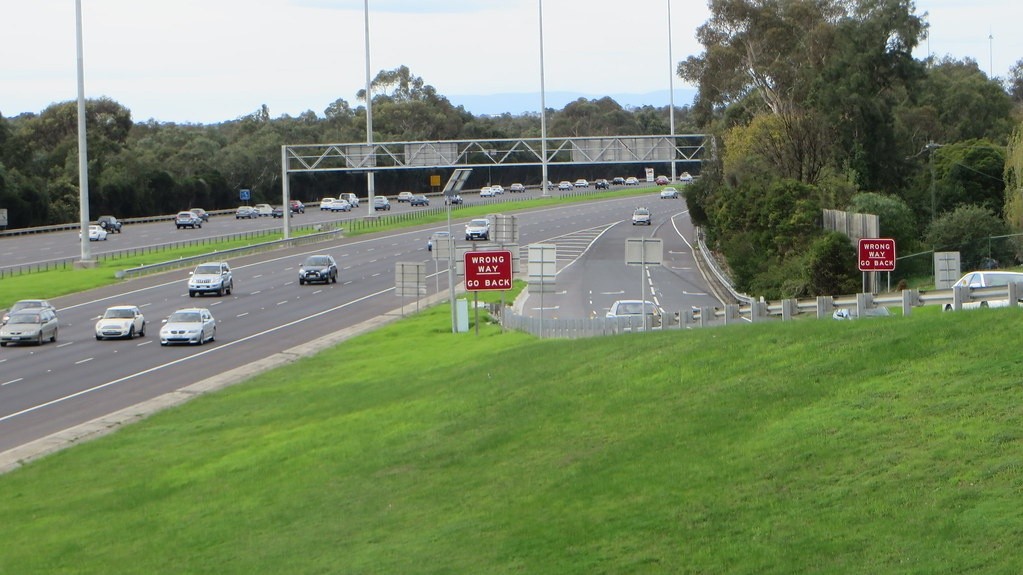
[187,261,234,297]
[98,216,122,233]
[176,211,201,229]
[463,218,492,240]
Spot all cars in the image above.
[445,194,464,204]
[253,204,275,217]
[539,181,554,191]
[289,200,305,214]
[374,196,391,211]
[319,193,360,212]
[654,175,670,186]
[510,183,526,193]
[410,194,430,206]
[558,181,574,191]
[678,172,694,183]
[298,255,339,283]
[272,206,294,219]
[830,303,896,319]
[595,179,610,189]
[158,307,217,345]
[0,306,60,347]
[480,184,505,197]
[612,177,640,185]
[427,231,456,251]
[236,206,258,219]
[938,270,1023,311]
[660,186,680,200]
[575,178,589,188]
[190,208,210,222]
[631,207,652,226]
[78,225,108,242]
[94,306,146,339]
[398,192,414,203]
[2,299,58,327]
[605,301,668,334]
